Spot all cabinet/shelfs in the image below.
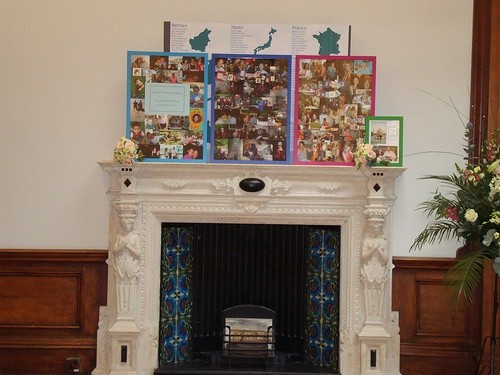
[0,248,110,375]
[392,254,494,375]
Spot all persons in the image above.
[126,52,399,166]
